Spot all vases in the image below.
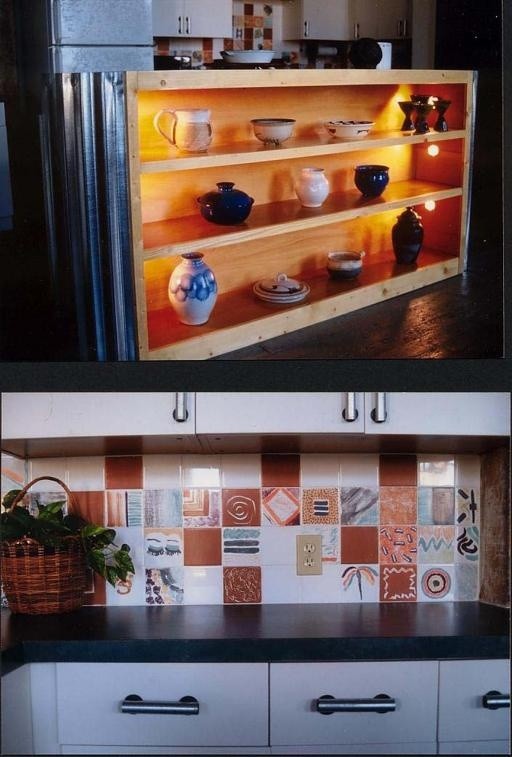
[354,165,390,199]
[326,249,365,280]
[398,92,450,135]
[294,167,330,208]
[167,253,218,325]
[392,207,425,265]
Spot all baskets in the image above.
[0,476,87,615]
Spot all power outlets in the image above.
[296,535,323,575]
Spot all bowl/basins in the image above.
[349,37,382,69]
[154,55,194,71]
[219,49,278,63]
[325,119,375,139]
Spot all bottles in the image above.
[168,165,423,327]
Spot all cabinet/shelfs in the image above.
[0,658,512,757]
[349,0,412,41]
[1,391,204,458]
[154,0,233,38]
[282,0,348,41]
[125,68,478,360]
[195,392,512,455]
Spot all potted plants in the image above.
[0,475,134,616]
